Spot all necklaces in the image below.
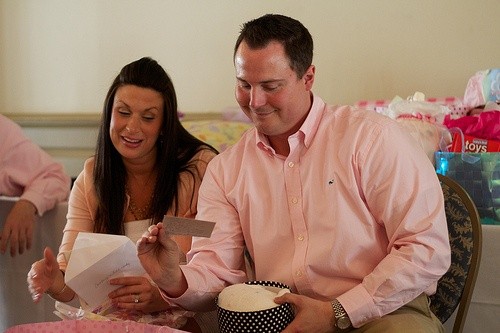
[124,190,153,220]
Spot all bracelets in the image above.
[45,269,67,297]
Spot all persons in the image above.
[0,114,71,257]
[135,15,451,333]
[26,57,220,333]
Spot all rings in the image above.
[134,294,138,304]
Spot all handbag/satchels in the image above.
[434,126,500,226]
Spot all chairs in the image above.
[430,173,483,333]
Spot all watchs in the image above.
[331,299,352,333]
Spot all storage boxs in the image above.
[214,280,295,333]
